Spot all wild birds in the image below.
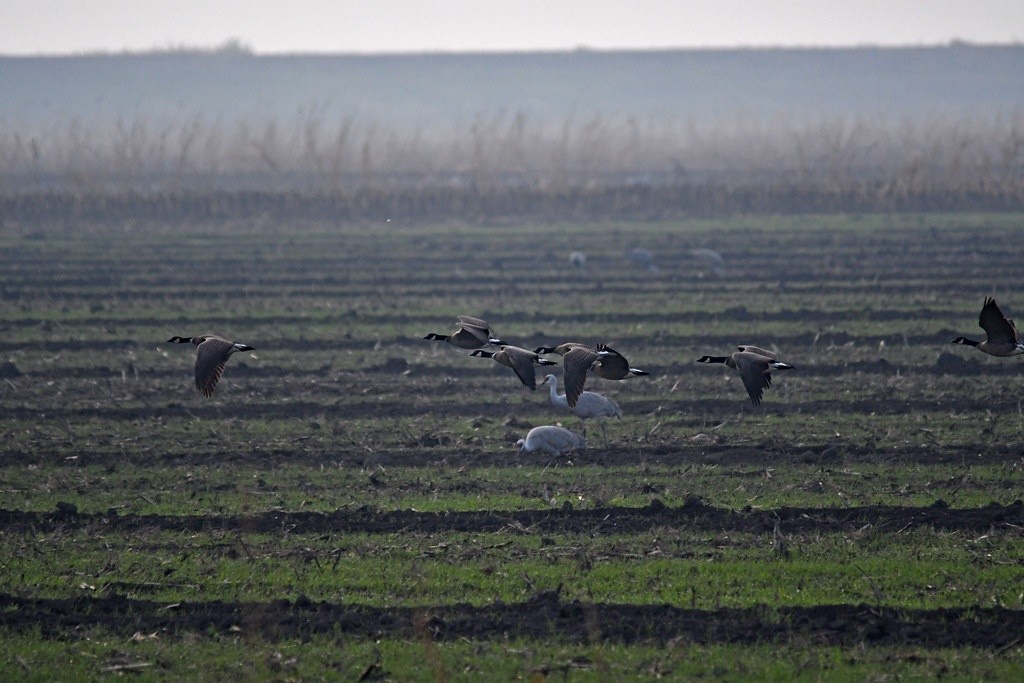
[950,296,1024,357]
[422,314,650,409]
[696,344,796,406]
[515,374,624,456]
[164,333,258,399]
